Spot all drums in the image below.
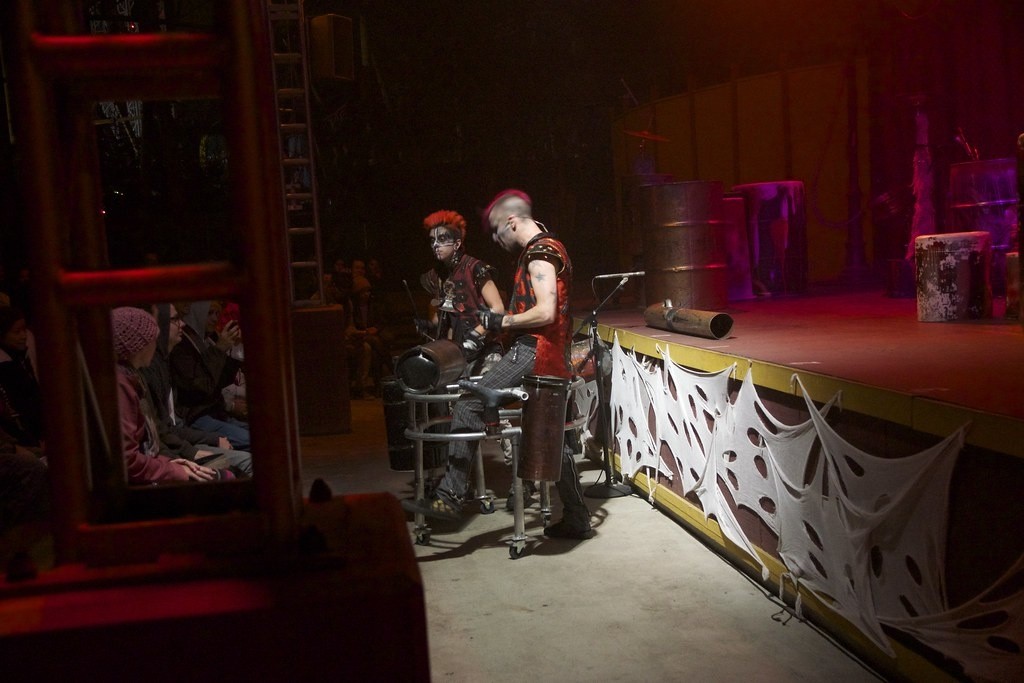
[396,338,466,392]
[516,373,572,483]
[379,374,433,472]
[570,335,611,385]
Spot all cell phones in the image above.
[228,321,238,330]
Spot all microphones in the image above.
[595,271,645,278]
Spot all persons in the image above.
[0,249,250,510]
[412,209,536,510]
[401,189,593,539]
[311,250,394,400]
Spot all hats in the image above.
[112,306,160,361]
[352,277,372,293]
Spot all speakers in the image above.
[292,297,352,436]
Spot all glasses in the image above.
[170,314,180,322]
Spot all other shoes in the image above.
[401,492,462,523]
[544,521,592,539]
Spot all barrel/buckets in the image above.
[734,180,809,299]
[718,194,755,303]
[640,178,729,311]
[915,230,992,323]
[950,157,1019,298]
